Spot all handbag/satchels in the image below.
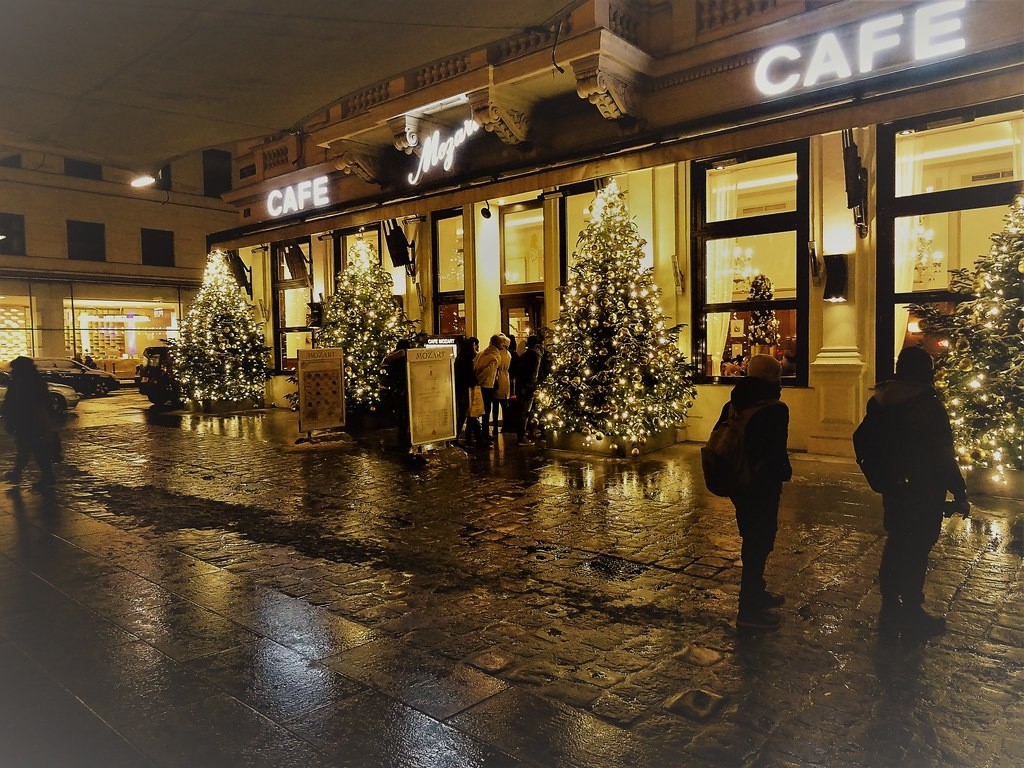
[468,385,485,418]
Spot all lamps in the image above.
[732,245,758,294]
[252,244,269,255]
[403,212,426,227]
[437,229,465,282]
[481,199,491,218]
[318,229,338,242]
[913,185,944,284]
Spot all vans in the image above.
[140,347,177,404]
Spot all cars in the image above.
[0,370,80,418]
[0,357,120,397]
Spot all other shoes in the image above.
[33,475,57,488]
[736,609,779,628]
[6,471,22,482]
[518,439,536,446]
[400,454,426,466]
[759,589,785,606]
[878,593,946,630]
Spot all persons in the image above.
[701,354,794,629]
[388,329,551,440]
[0,355,60,494]
[851,345,972,630]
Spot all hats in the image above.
[499,333,510,347]
[10,356,33,368]
[748,354,783,382]
[896,346,933,383]
[490,335,504,347]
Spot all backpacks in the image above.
[700,402,767,497]
[853,411,914,494]
